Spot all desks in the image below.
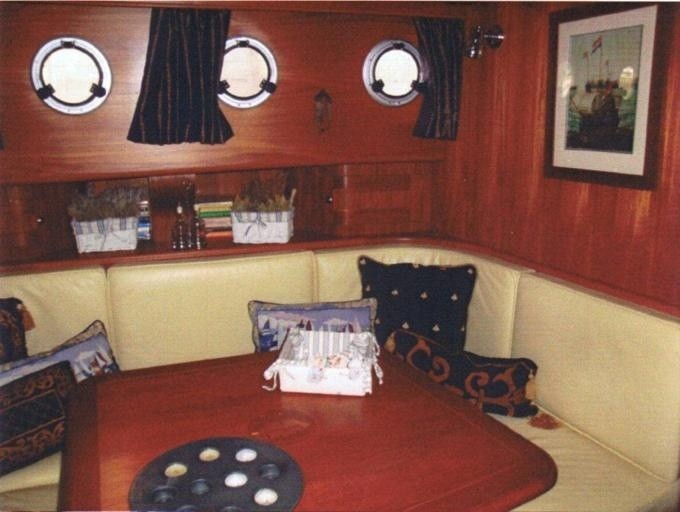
[58,345,558,511]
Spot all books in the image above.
[136,199,236,240]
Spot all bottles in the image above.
[136,199,153,241]
[171,199,208,251]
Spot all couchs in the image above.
[1,236,680,512]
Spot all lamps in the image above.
[466,23,506,63]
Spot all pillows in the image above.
[358,256,477,349]
[382,326,542,420]
[1,319,122,478]
[247,297,379,353]
[1,296,35,363]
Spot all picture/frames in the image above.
[540,1,675,189]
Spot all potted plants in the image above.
[69,190,141,256]
[229,194,296,245]
